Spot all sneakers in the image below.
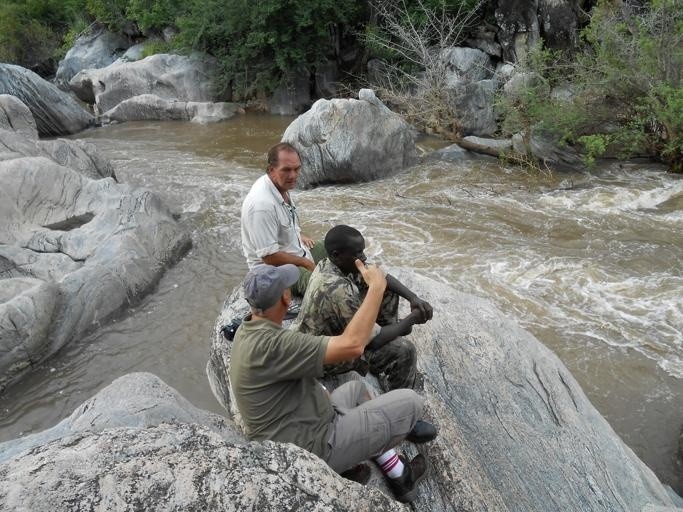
[340,465,371,484]
[406,420,437,444]
[388,453,429,503]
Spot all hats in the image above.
[243,264,300,312]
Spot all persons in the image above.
[231,260,437,503]
[240,141,330,297]
[298,225,436,444]
